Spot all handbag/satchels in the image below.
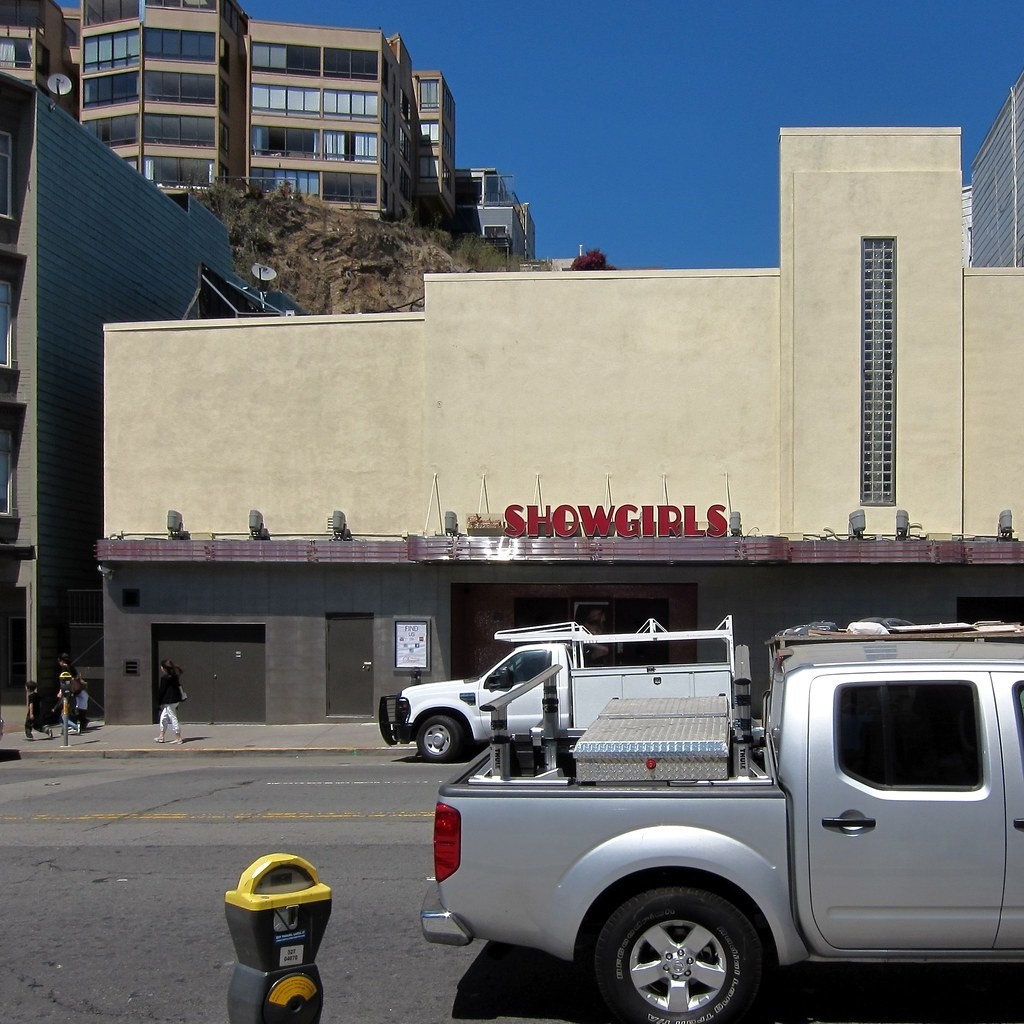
[179,685,187,701]
[70,678,83,696]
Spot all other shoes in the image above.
[77,724,80,734]
[25,737,33,740]
[49,729,52,738]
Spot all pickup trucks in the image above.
[421,639,1023,1023]
[379,616,737,762]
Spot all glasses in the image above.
[160,667,161,669]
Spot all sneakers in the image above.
[154,737,164,742]
[169,738,183,744]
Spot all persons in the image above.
[23,681,53,740]
[56,653,81,734]
[154,659,188,744]
[52,689,81,736]
[76,679,89,733]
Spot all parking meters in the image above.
[224,852,332,1024]
[58,671,73,745]
